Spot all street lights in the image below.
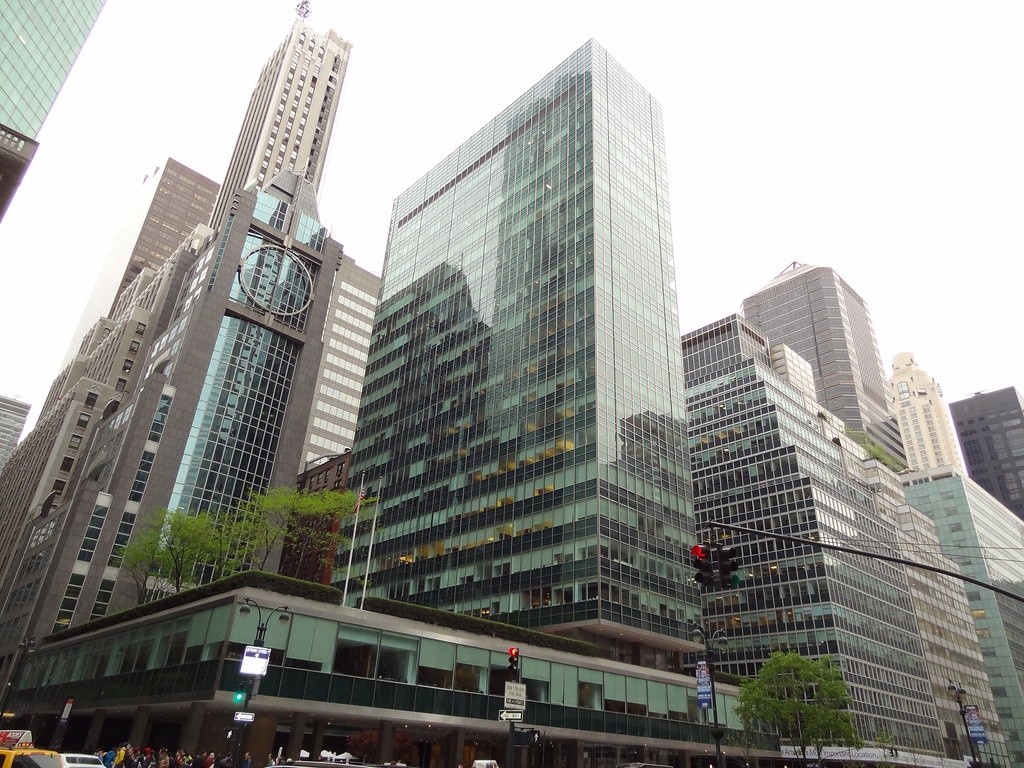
[232,596,290,768]
[691,622,728,768]
[947,678,977,768]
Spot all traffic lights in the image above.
[235,688,243,702]
[508,647,518,679]
[528,730,540,746]
[693,541,713,586]
[717,547,740,589]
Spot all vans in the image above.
[0,729,63,768]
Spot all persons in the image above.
[49,739,64,753]
[243,752,252,768]
[267,754,285,766]
[94,743,233,768]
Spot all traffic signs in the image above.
[233,712,255,722]
[498,710,524,723]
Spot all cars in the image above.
[59,754,106,768]
